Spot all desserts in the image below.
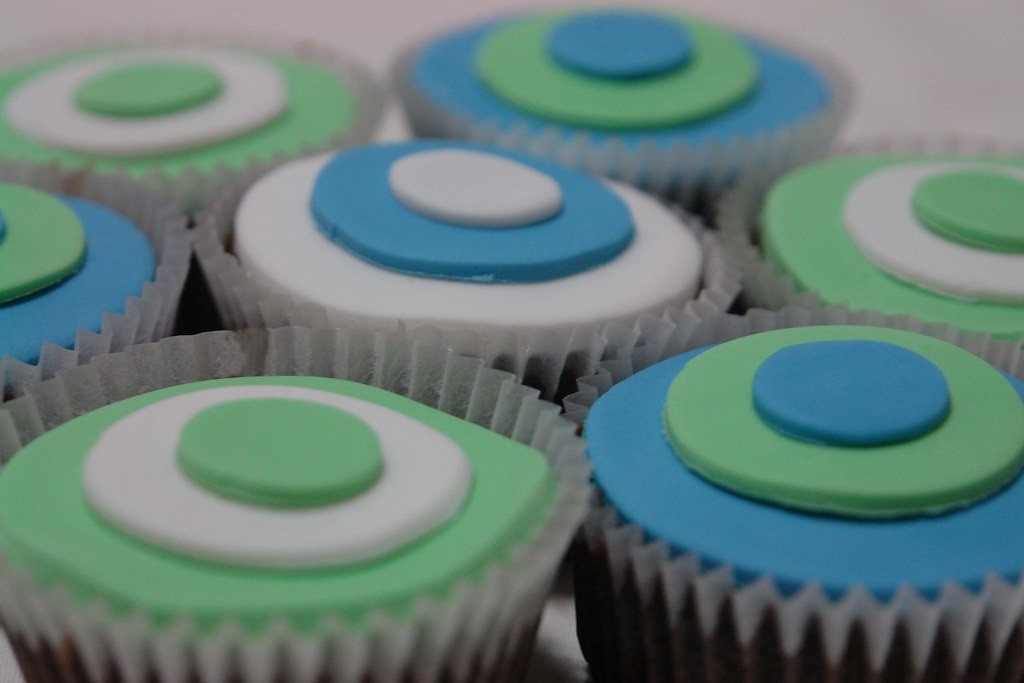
[0,10,1024,683]
